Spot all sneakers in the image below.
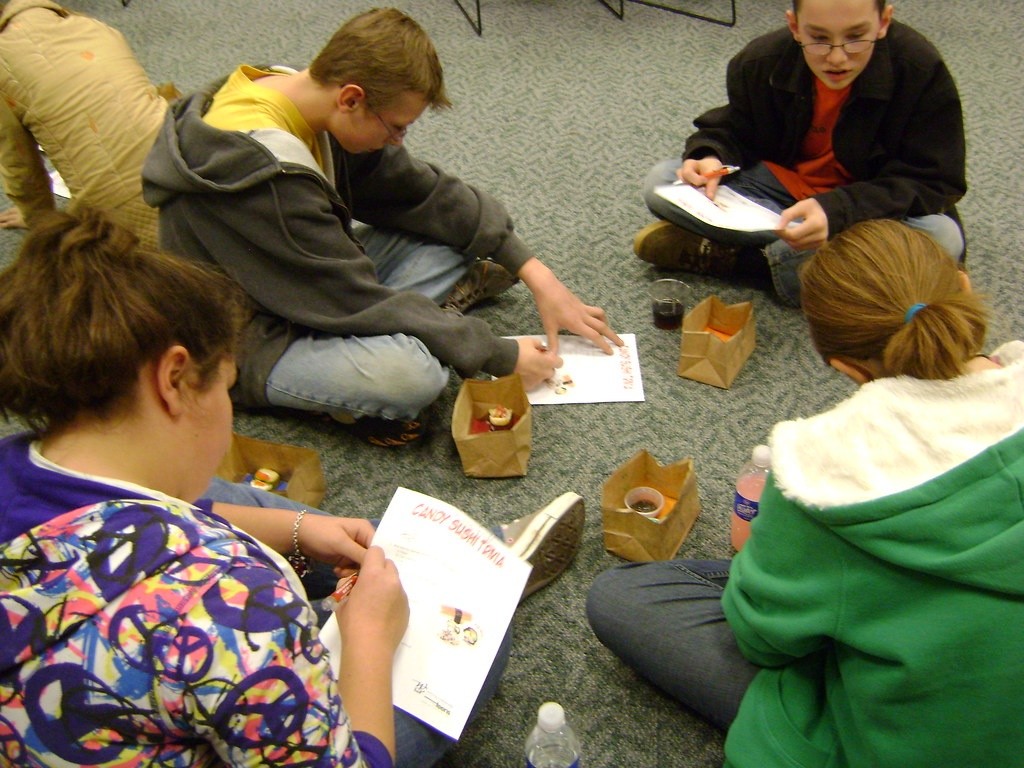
[311,399,428,448]
[632,220,744,283]
[439,257,519,318]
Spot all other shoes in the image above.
[499,492,586,605]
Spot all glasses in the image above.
[797,34,877,56]
[364,100,407,143]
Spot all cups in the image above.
[624,486,664,518]
[646,279,693,331]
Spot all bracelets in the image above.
[293,510,307,561]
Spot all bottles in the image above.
[731,444,770,552]
[524,702,581,768]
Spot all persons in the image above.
[632,0,967,310]
[584,219,1024,768]
[0,0,623,447]
[1,203,584,768]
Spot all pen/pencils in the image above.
[322,572,358,611]
[673,166,741,186]
[538,345,547,352]
